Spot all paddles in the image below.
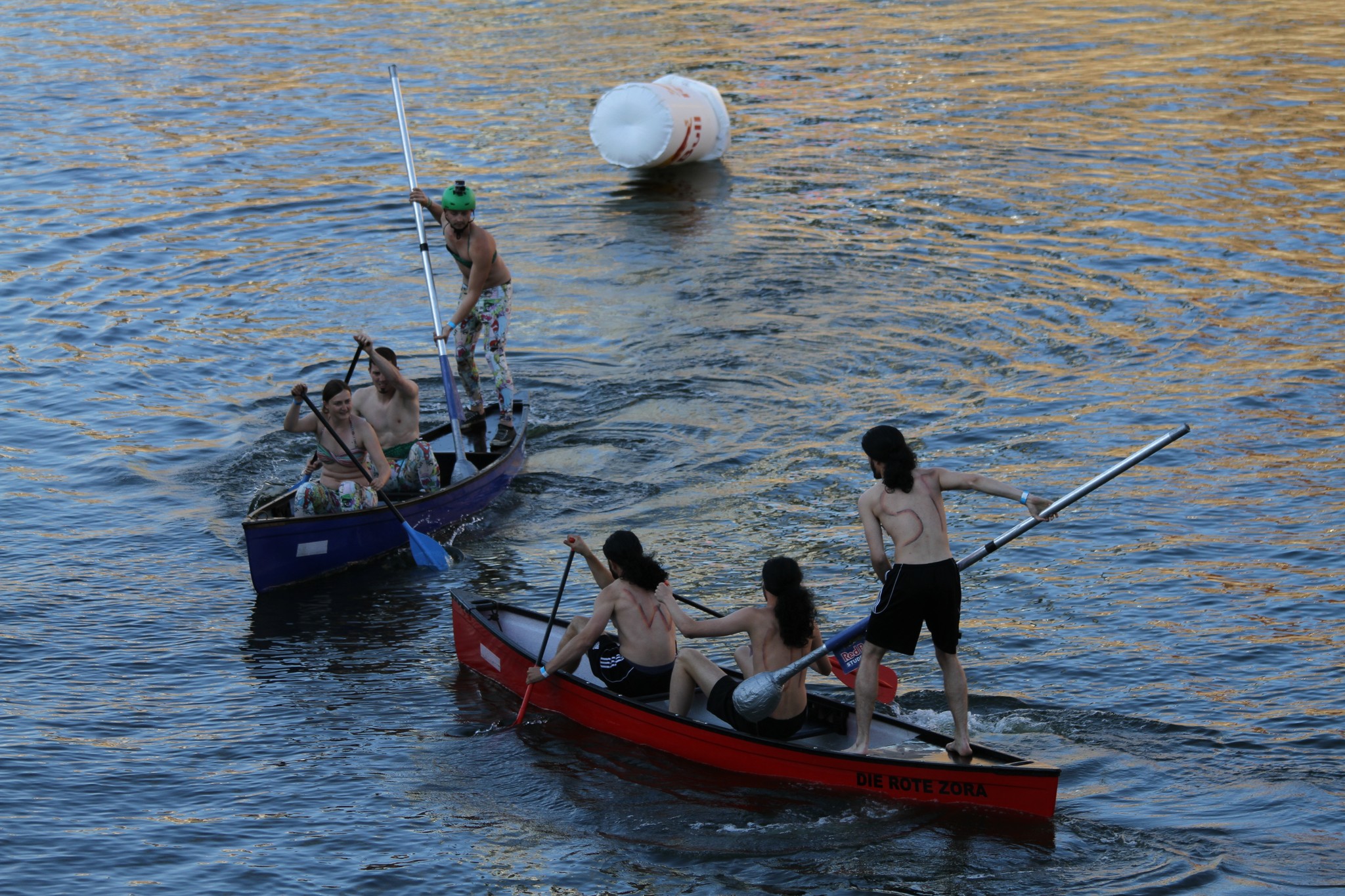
[296,388,454,572]
[668,592,900,709]
[513,539,575,729]
[290,333,373,492]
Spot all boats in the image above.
[447,586,1064,819]
[239,388,533,594]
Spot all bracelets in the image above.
[1020,491,1030,504]
[421,197,432,209]
[540,666,551,678]
[293,398,304,405]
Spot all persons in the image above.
[282,379,392,518]
[409,180,519,448]
[836,425,1058,753]
[305,332,443,496]
[527,531,678,699]
[654,555,833,735]
